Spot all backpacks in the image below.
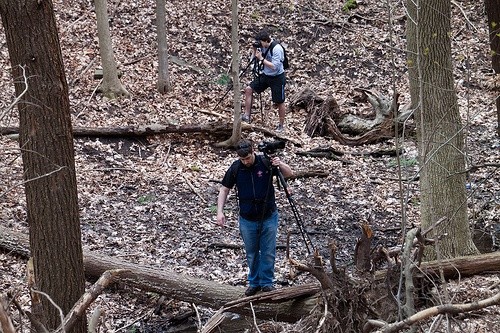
[270,41,289,70]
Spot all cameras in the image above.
[258,140,285,155]
[252,41,262,48]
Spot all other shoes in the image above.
[242,114,251,122]
[245,285,260,296]
[263,286,275,291]
[277,125,282,130]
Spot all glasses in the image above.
[236,143,251,150]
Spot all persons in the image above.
[216,142,293,296]
[241,31,286,132]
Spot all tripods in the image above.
[251,167,315,271]
[212,57,264,124]
